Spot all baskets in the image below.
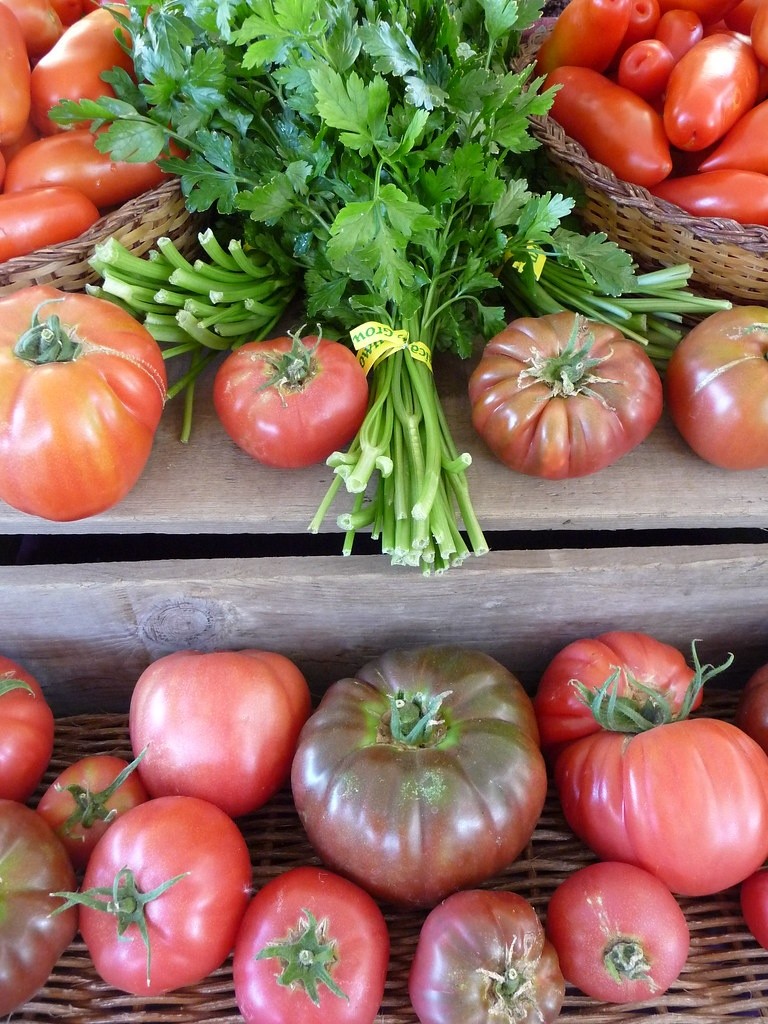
[507,16,768,328]
[0,173,236,307]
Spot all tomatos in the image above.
[214,0,768,528]
[0,634,766,1024]
[1,0,172,523]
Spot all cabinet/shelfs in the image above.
[0,6,768,1020]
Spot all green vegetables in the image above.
[56,0,731,576]
